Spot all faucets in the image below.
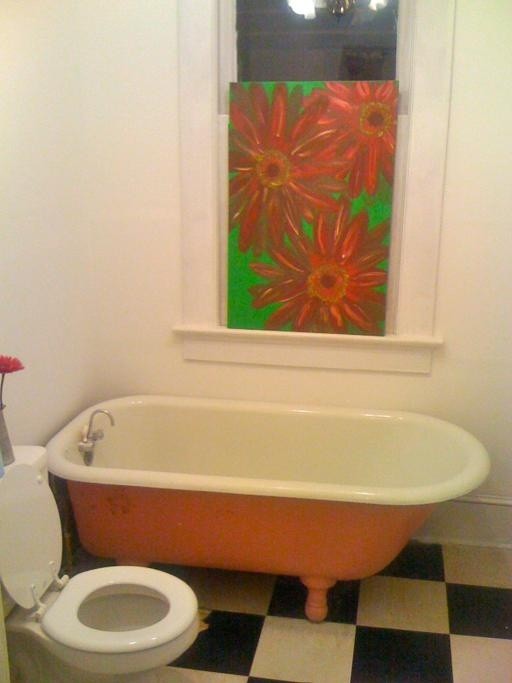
[85,407,115,438]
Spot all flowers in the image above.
[0,353,26,404]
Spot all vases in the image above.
[0,402,16,464]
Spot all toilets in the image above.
[0,443,202,682]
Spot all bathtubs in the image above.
[48,397,492,580]
